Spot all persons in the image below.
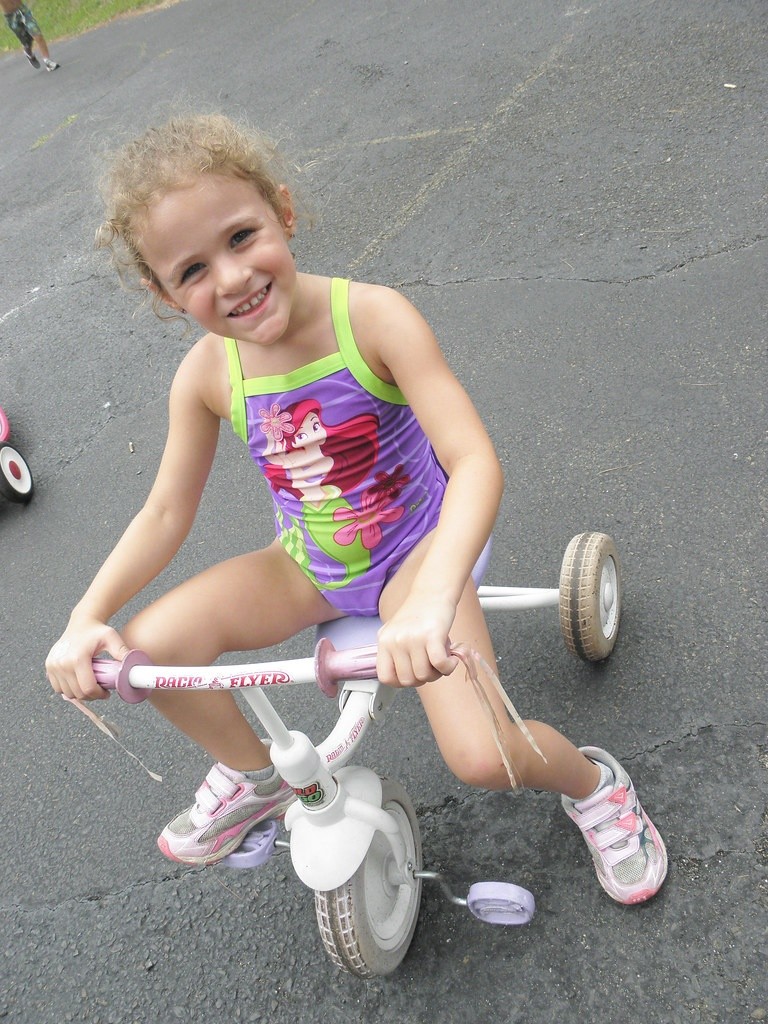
[46,114,669,906]
[0,0,60,72]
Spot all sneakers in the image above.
[43,59,59,71]
[558,746,668,907]
[155,737,289,867]
[25,51,40,69]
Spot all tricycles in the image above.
[89,531,625,980]
